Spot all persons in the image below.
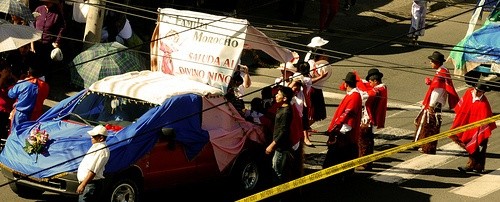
[318,0,339,38]
[408,51,460,154]
[76,125,110,202]
[405,0,427,45]
[321,69,387,202]
[0,0,70,136]
[224,37,329,202]
[64,0,101,59]
[450,70,497,173]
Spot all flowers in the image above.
[24,126,49,156]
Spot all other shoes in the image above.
[355,166,372,171]
[304,141,315,147]
[457,166,473,173]
[418,148,425,153]
[472,168,486,172]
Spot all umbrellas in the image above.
[73,43,148,93]
[0,24,43,51]
[0,0,33,21]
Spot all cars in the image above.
[1,9,302,202]
[459,0,500,91]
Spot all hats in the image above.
[342,72,359,82]
[366,68,384,81]
[307,36,329,47]
[276,61,297,72]
[313,59,332,81]
[475,84,487,93]
[428,51,446,63]
[87,125,108,136]
